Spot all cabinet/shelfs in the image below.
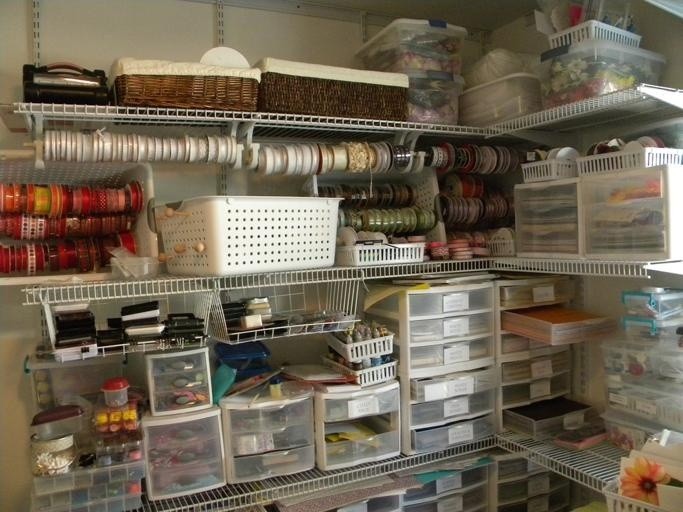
[490,82,682,510]
[3,105,496,510]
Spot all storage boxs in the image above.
[624,317,681,349]
[513,178,581,259]
[623,286,683,322]
[457,72,539,125]
[27,433,144,511]
[495,274,617,438]
[537,41,665,108]
[362,284,499,451]
[601,335,682,386]
[354,17,468,81]
[312,378,401,472]
[600,371,681,430]
[140,403,226,500]
[601,409,661,452]
[402,78,459,124]
[219,392,316,485]
[146,348,213,411]
[577,163,682,262]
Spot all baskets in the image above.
[318,350,399,388]
[336,241,426,268]
[250,57,410,124]
[321,326,394,364]
[0,158,158,287]
[105,56,261,112]
[574,146,682,177]
[601,474,669,511]
[298,166,447,247]
[547,19,642,50]
[519,160,577,184]
[152,192,344,276]
